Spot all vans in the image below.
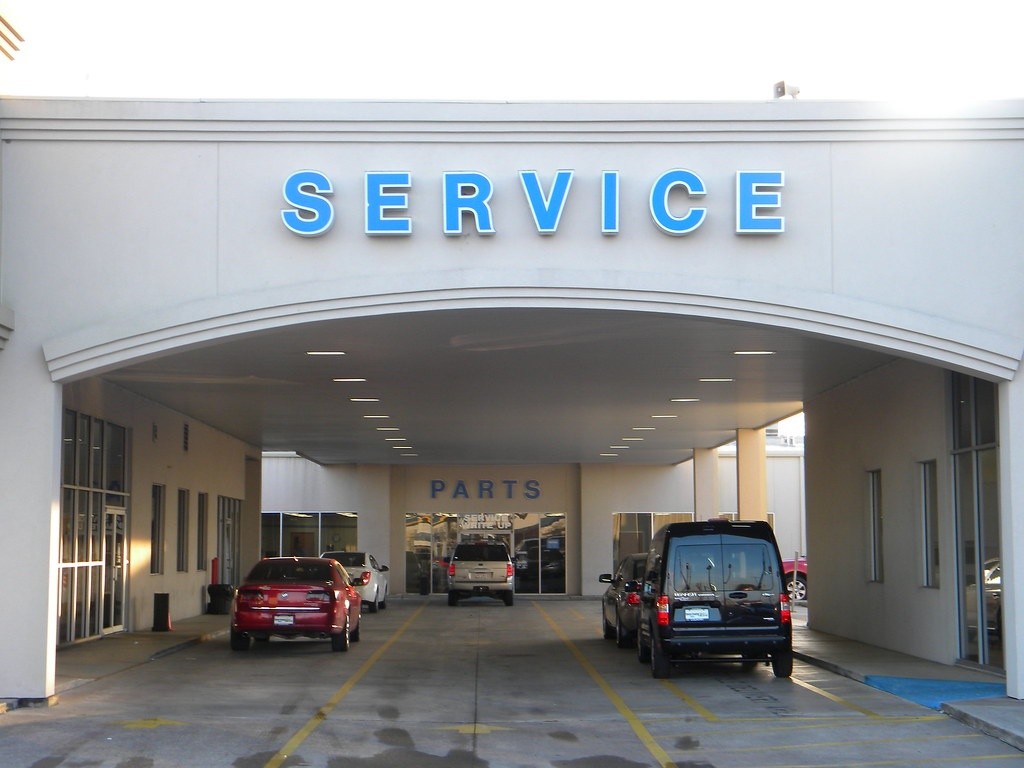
[624,518,794,678]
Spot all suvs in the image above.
[442,538,520,608]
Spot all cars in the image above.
[319,551,389,613]
[407,551,450,593]
[966,557,1003,642]
[781,558,807,602]
[599,552,649,652]
[231,557,364,653]
[515,535,567,592]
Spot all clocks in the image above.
[332,533,342,543]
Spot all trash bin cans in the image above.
[207,584,236,614]
